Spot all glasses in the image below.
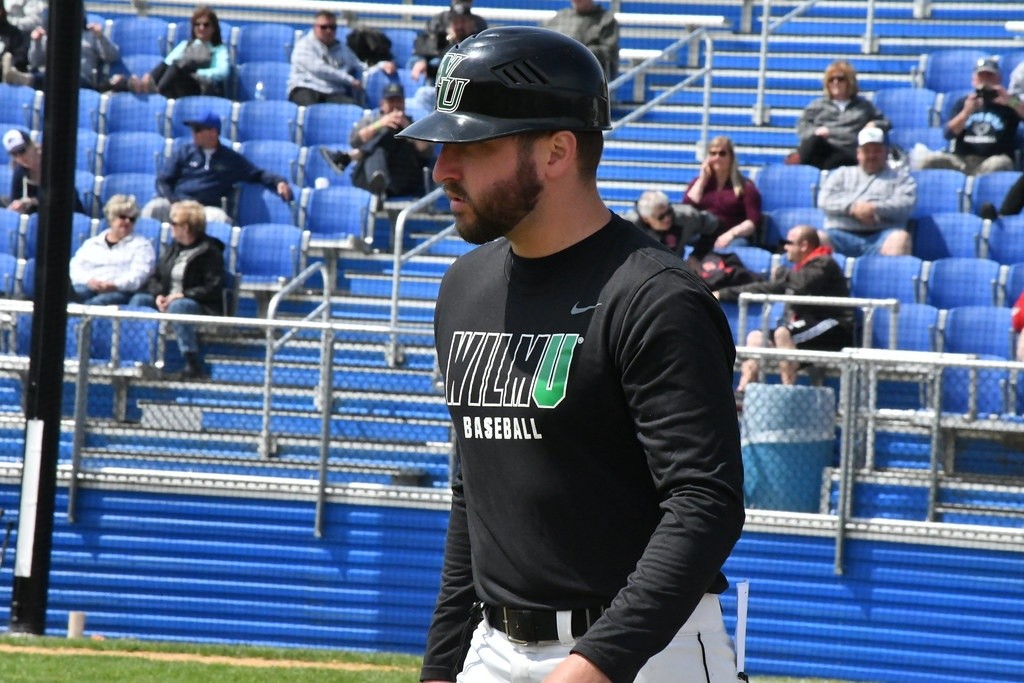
[316,23,339,31]
[657,205,674,221]
[779,238,794,246]
[194,21,213,27]
[119,215,136,223]
[8,146,28,154]
[828,76,846,81]
[709,150,730,157]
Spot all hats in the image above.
[381,83,405,99]
[182,110,221,130]
[976,60,1000,76]
[2,129,31,154]
[857,127,885,146]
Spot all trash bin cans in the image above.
[741,381,836,512]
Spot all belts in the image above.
[474,569,730,647]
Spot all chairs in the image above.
[0,11,447,459]
[713,46,1024,422]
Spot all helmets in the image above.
[392,26,612,145]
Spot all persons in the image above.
[784,61,894,170]
[816,126,918,257]
[979,175,1024,221]
[1012,290,1024,362]
[129,199,225,380]
[287,11,395,106]
[321,82,437,211]
[1008,62,1024,102]
[920,60,1024,175]
[623,190,720,278]
[0,0,128,92]
[393,27,745,683]
[682,137,761,251]
[711,226,854,411]
[0,129,85,215]
[546,0,619,82]
[68,194,156,306]
[131,6,230,99]
[412,0,487,121]
[139,111,293,226]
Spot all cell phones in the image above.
[975,89,997,98]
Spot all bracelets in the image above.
[1009,97,1018,108]
